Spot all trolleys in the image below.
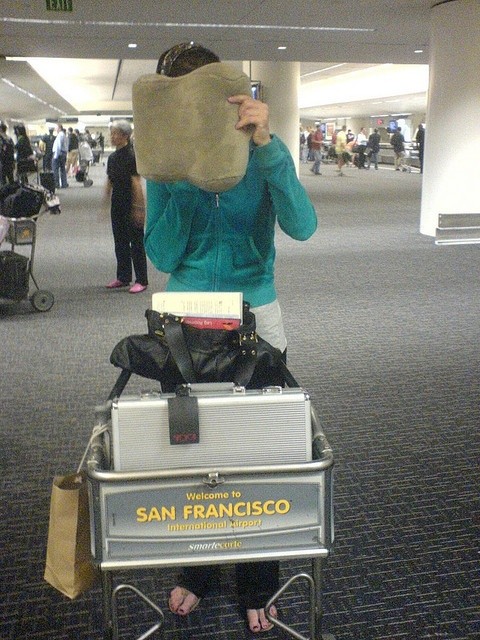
[82,358,338,638]
[0,204,62,315]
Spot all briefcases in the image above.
[111,382,314,475]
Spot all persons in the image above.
[358,127,369,170]
[0,122,15,186]
[11,122,35,186]
[332,129,339,147]
[302,126,312,164]
[334,124,351,171]
[365,127,382,170]
[299,122,307,160]
[387,120,397,132]
[390,126,412,173]
[141,41,319,634]
[40,122,105,189]
[345,129,355,143]
[415,122,425,173]
[308,124,325,176]
[307,129,315,161]
[98,119,149,294]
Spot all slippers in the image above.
[107,280,128,290]
[129,283,147,293]
[247,604,277,633]
[169,586,202,615]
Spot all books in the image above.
[151,290,245,330]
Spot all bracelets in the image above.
[132,202,147,210]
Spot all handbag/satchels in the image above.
[42,425,109,600]
[109,299,287,390]
